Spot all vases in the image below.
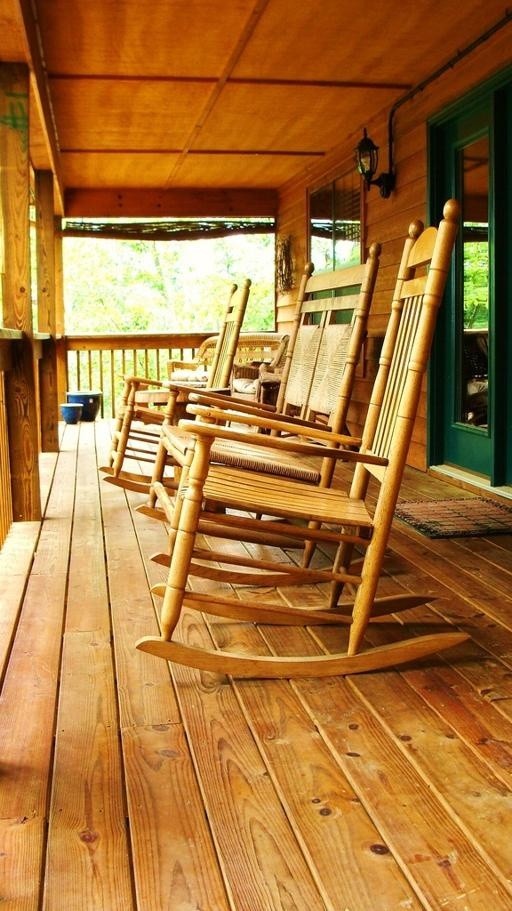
[60,403,83,424]
[66,392,102,422]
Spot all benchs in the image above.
[166,332,289,390]
[134,242,394,588]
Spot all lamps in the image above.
[355,126,394,198]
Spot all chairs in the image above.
[98,279,252,498]
[227,341,292,435]
[134,198,472,678]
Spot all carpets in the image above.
[394,496,512,538]
